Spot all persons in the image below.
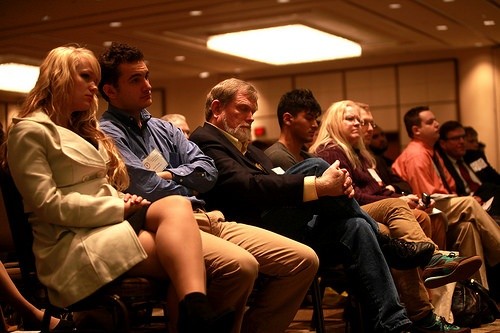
[1,42,319,332]
[161,77,500,333]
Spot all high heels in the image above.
[177,292,237,333]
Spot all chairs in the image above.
[0,158,354,333]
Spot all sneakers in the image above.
[410,316,471,333]
[376,234,435,270]
[419,253,482,288]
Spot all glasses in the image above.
[360,122,376,127]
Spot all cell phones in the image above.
[422,193,430,209]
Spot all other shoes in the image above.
[51,319,75,333]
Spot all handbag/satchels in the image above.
[451,279,499,328]
